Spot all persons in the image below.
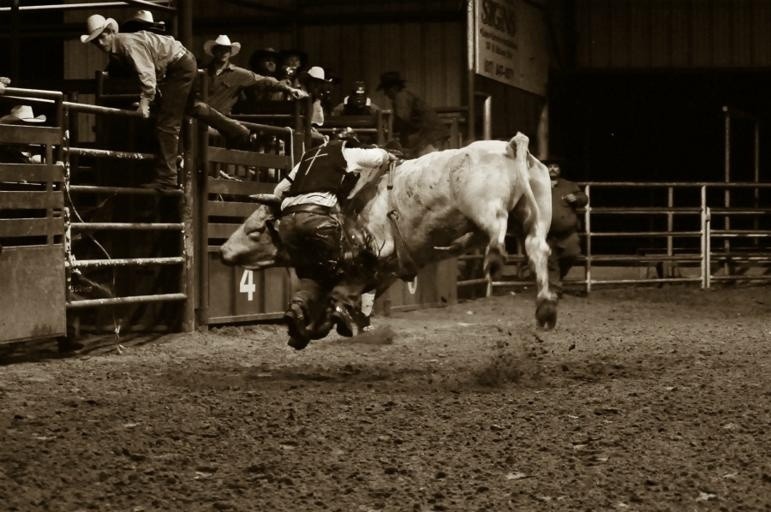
[274,127,392,340]
[121,9,441,171]
[80,12,256,192]
[544,161,589,298]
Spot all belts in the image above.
[280,205,331,216]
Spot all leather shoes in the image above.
[332,305,353,338]
[286,309,310,344]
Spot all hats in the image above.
[333,125,359,143]
[81,14,120,44]
[127,11,165,25]
[0,104,46,124]
[204,34,240,57]
[256,48,280,67]
[540,155,564,167]
[350,82,367,106]
[304,67,333,81]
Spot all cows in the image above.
[220,132,559,335]
